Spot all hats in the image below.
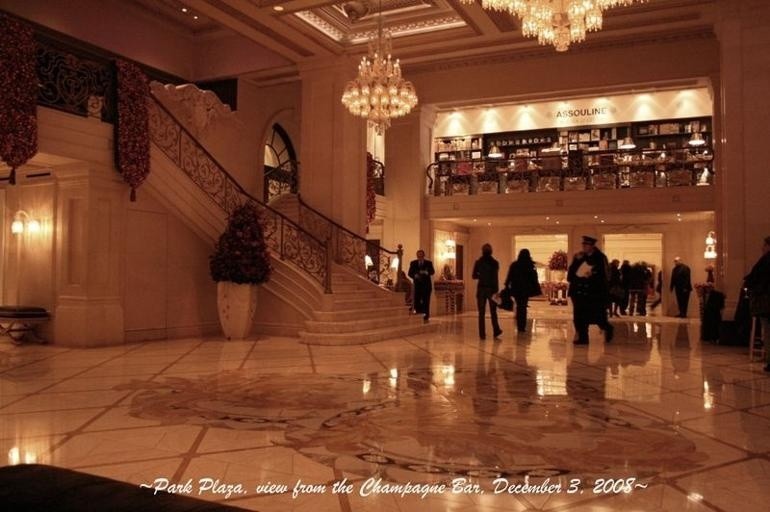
[581,236,597,247]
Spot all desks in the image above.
[434,279,468,315]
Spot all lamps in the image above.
[458,0,646,54]
[703,229,717,245]
[338,1,420,133]
[442,240,456,248]
[703,245,717,259]
[8,209,41,236]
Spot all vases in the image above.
[214,280,262,337]
[549,270,569,284]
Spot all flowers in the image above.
[549,250,570,270]
[205,197,273,287]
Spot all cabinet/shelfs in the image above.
[436,133,482,175]
[560,115,714,153]
[482,126,560,159]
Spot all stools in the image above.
[748,312,769,365]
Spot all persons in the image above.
[505,249,542,332]
[472,244,503,340]
[669,256,692,317]
[370,265,413,306]
[409,250,435,322]
[440,264,454,281]
[742,236,770,372]
[608,259,664,318]
[566,236,615,345]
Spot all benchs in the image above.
[0,304,51,347]
